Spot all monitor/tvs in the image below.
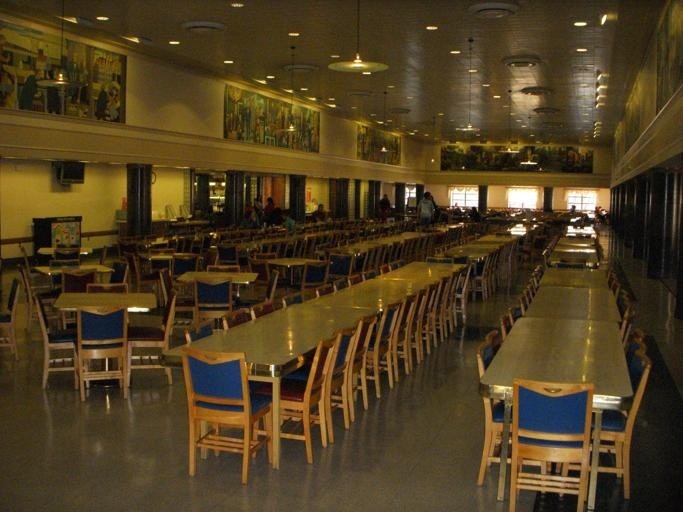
[60,161,84,184]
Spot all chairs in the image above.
[284,329,357,444]
[159,269,195,312]
[347,317,378,423]
[347,275,362,284]
[281,293,304,307]
[184,323,216,341]
[126,216,599,260]
[0,277,23,361]
[179,348,272,484]
[33,293,77,390]
[509,378,594,512]
[314,284,333,299]
[473,253,500,301]
[368,304,402,395]
[193,277,232,332]
[327,332,358,442]
[74,304,128,402]
[559,351,652,499]
[109,261,130,292]
[219,309,255,329]
[238,270,280,309]
[422,270,476,354]
[129,290,178,385]
[251,340,337,464]
[485,329,502,351]
[625,339,647,372]
[475,343,551,493]
[401,294,420,374]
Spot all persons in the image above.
[596,207,608,224]
[544,204,553,213]
[268,207,283,225]
[245,201,255,219]
[469,207,480,222]
[417,192,434,225]
[254,195,263,218]
[97,81,121,122]
[94,50,122,84]
[378,205,388,219]
[569,205,576,213]
[34,49,49,80]
[312,204,327,222]
[280,212,295,234]
[429,196,435,223]
[435,206,443,222]
[47,72,63,115]
[20,69,40,110]
[379,194,390,208]
[265,198,274,215]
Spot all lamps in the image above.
[52,0,74,86]
[522,116,540,166]
[327,0,388,74]
[455,32,482,133]
[497,91,523,155]
[379,91,391,153]
[283,34,302,133]
